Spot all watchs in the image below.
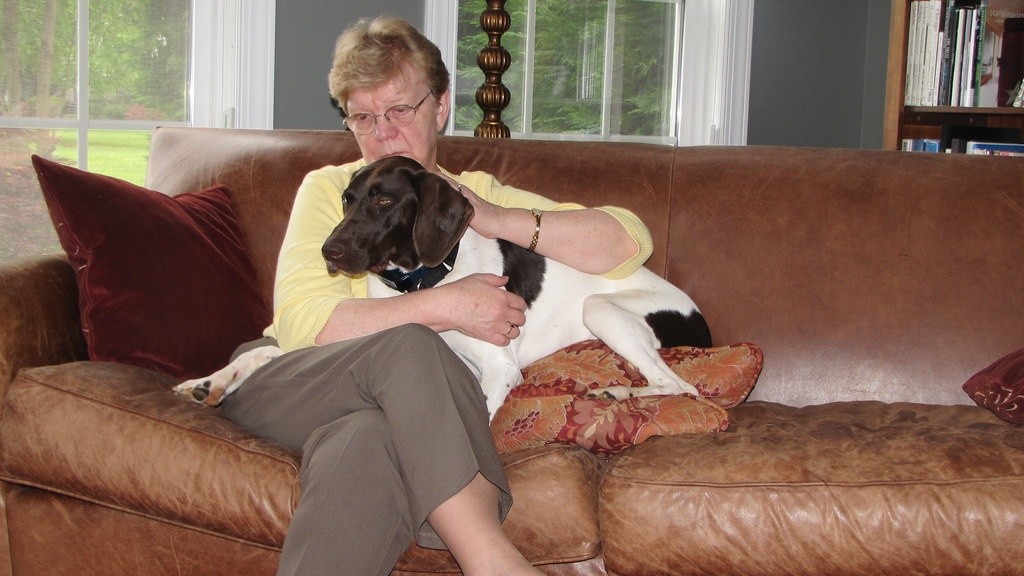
[527,207,543,252]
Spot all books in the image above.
[903,0,1024,107]
[902,139,1024,155]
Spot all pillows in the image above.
[486,340,764,455]
[961,349,1024,427]
[30,154,270,381]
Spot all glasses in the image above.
[343,88,432,135]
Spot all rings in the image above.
[504,324,514,336]
[458,183,462,193]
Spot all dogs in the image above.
[173,155,712,428]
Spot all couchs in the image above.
[0,124,1024,576]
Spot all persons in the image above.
[221,16,653,576]
